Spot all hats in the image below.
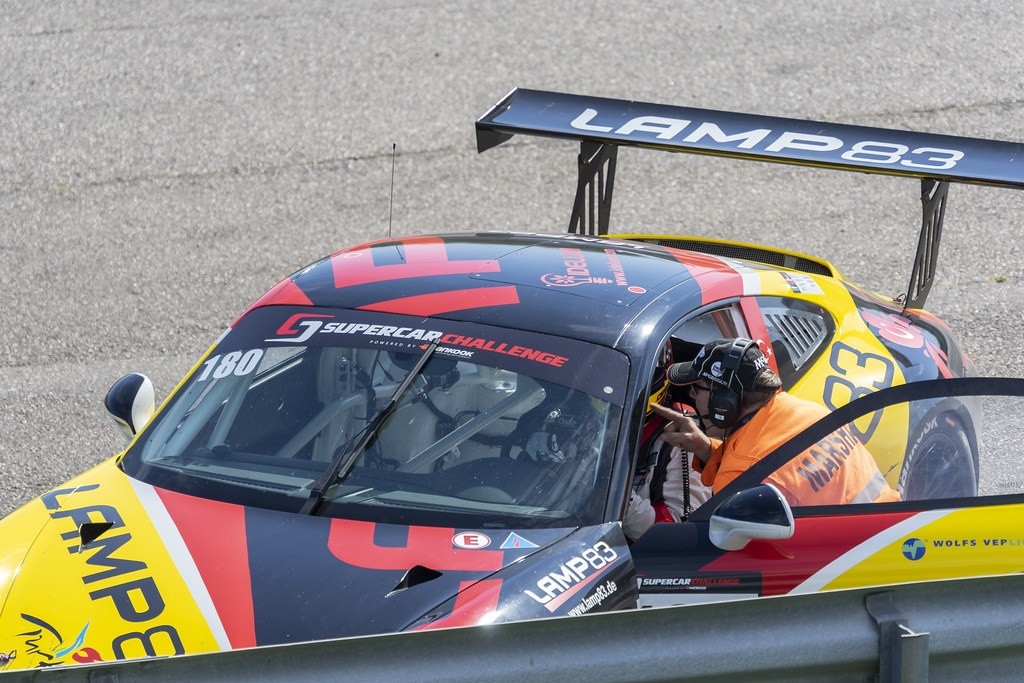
[667,338,780,393]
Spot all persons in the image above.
[650,337,903,511]
[585,337,713,543]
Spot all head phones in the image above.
[708,337,758,430]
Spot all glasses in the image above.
[690,383,711,394]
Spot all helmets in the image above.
[587,338,675,420]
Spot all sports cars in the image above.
[0,83,1023,677]
